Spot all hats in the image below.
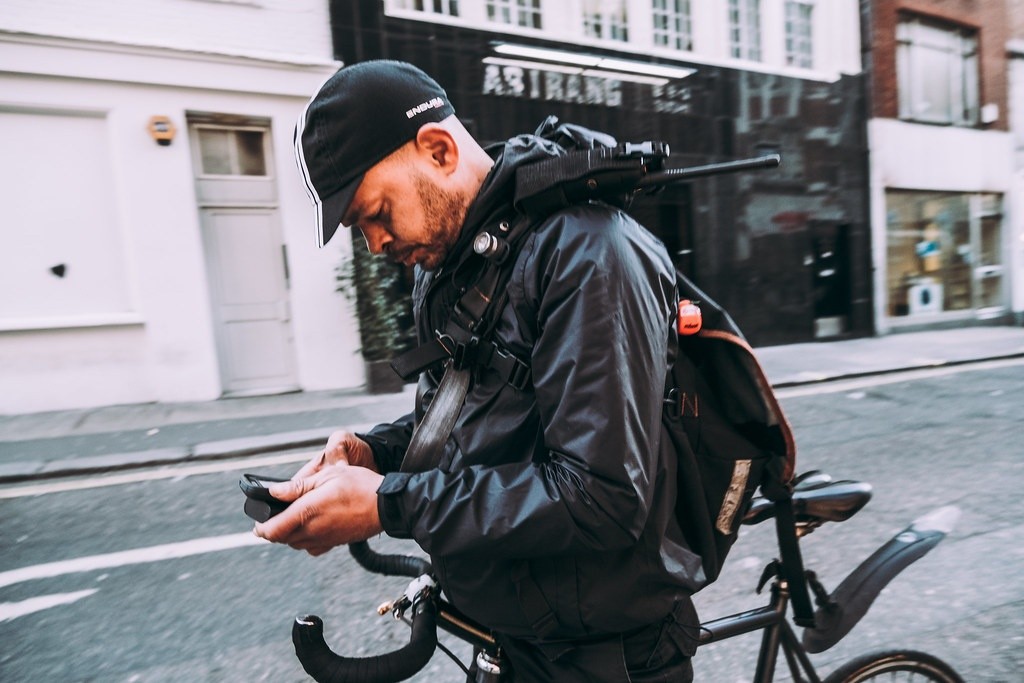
[292,57,455,250]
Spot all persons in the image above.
[253,59,700,683]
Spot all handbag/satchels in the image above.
[503,199,770,589]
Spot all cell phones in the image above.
[239,473,292,504]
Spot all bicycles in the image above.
[292,468,967,683]
